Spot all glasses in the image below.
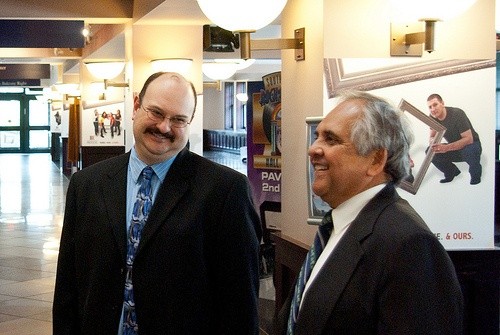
[140,103,190,128]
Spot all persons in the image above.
[268,89,466,335]
[51,74,262,335]
[425,94,482,185]
[93,109,122,139]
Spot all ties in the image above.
[286,211,333,335]
[121,167,154,335]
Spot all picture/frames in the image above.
[391,98,446,196]
[324,58,497,98]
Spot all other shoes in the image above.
[470,175,481,185]
[440,164,461,183]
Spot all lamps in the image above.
[389,1,477,58]
[203,60,239,92]
[84,62,129,91]
[151,58,193,81]
[197,0,305,60]
